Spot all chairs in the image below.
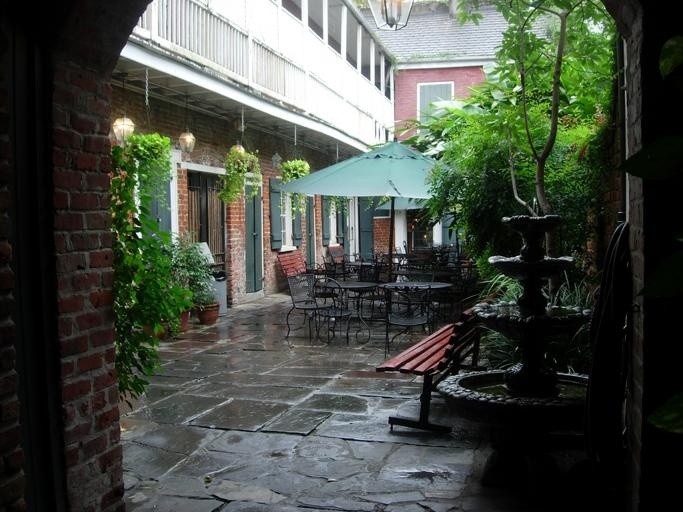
[284,241,513,347]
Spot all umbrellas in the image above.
[372,197,436,252]
[269,136,454,313]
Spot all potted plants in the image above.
[217,142,311,214]
[143,226,221,342]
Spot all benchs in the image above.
[376,289,504,436]
[276,250,311,293]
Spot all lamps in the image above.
[270,127,284,172]
[108,70,248,158]
[368,0,414,34]
